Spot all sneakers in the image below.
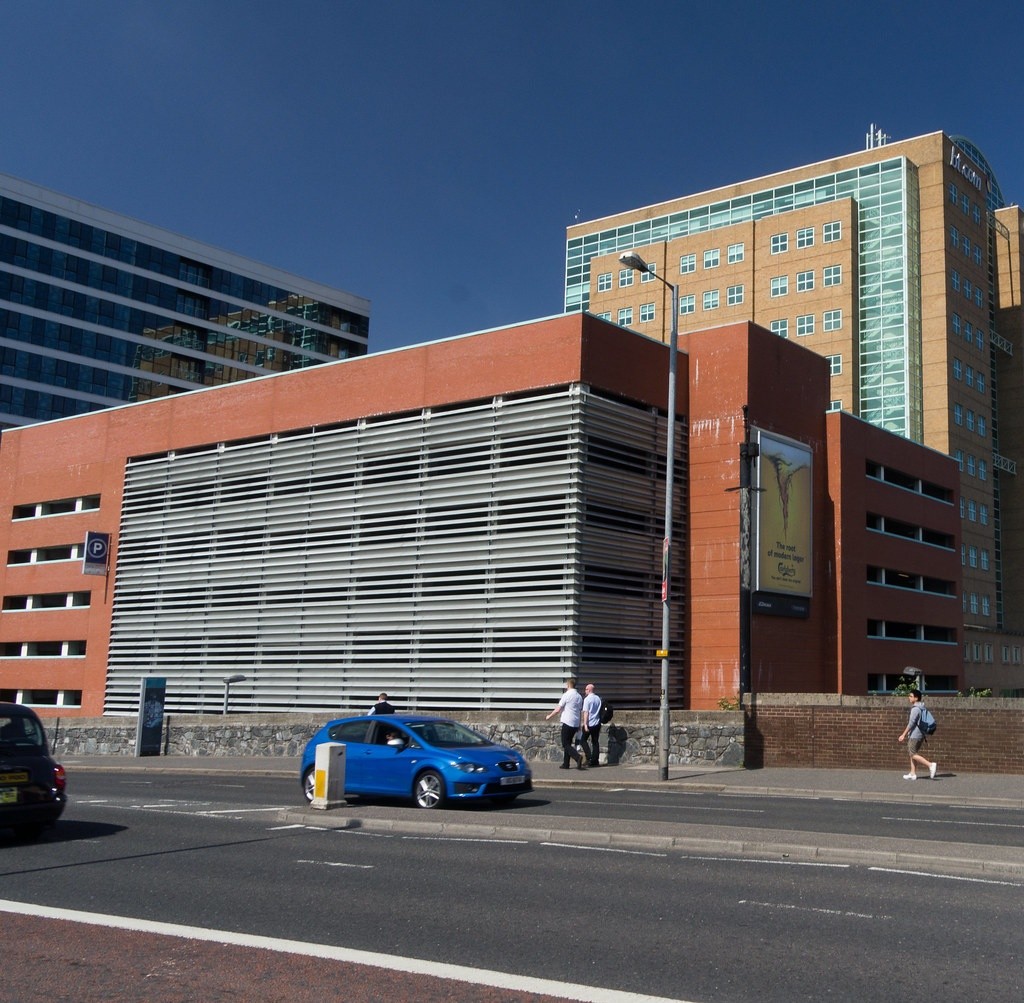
[930,763,936,779]
[903,773,916,780]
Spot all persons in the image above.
[546,678,582,769]
[898,689,936,780]
[367,693,395,715]
[582,683,601,767]
[385,728,406,744]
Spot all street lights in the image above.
[223,675,246,714]
[903,666,925,700]
[618,250,680,779]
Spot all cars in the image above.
[0,703,68,826]
[299,713,533,808]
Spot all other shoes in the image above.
[559,764,569,769]
[582,760,599,767]
[575,756,583,768]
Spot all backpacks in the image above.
[911,705,936,742]
[600,701,613,724]
